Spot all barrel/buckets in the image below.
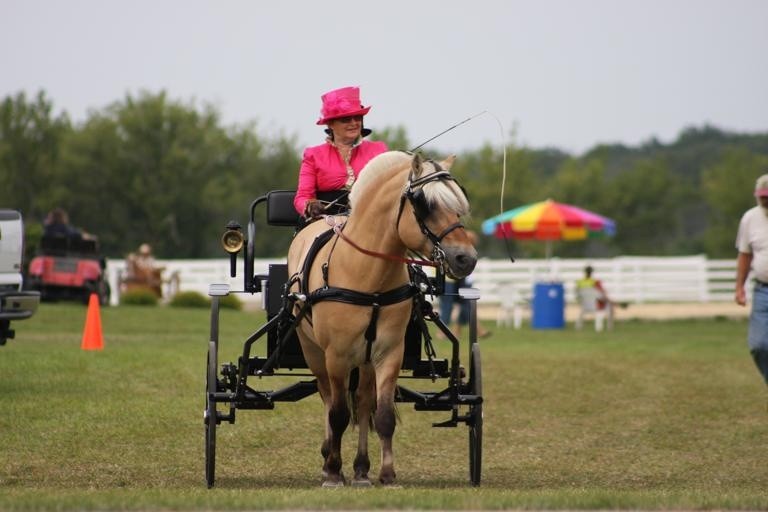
[438,275,475,323]
[530,282,567,330]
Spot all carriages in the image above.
[201,150,486,492]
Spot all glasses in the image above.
[340,115,362,123]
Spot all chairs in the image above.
[495,273,620,332]
[263,186,428,372]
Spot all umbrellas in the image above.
[482,198,616,285]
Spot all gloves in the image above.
[307,201,328,218]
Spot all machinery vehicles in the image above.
[29,229,112,306]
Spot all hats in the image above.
[754,175,768,198]
[316,87,370,125]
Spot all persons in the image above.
[576,266,629,311]
[436,274,492,340]
[293,87,388,221]
[734,174,768,385]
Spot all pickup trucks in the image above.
[0,209,41,348]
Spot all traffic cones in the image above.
[79,293,105,352]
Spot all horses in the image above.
[286,149,480,489]
